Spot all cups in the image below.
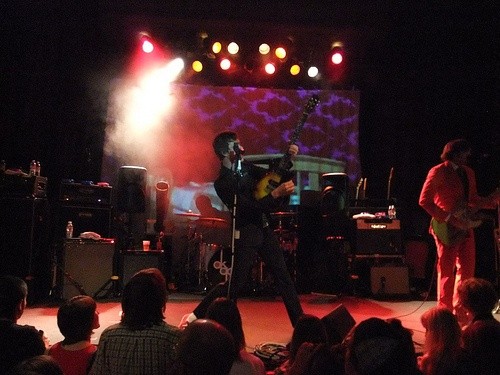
[143,241,150,251]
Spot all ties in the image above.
[457,166,470,203]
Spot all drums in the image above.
[270,213,297,233]
[203,246,232,283]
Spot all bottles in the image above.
[35,161,40,176]
[391,204,396,219]
[388,205,392,219]
[0,160,5,171]
[66,221,73,238]
[29,160,36,175]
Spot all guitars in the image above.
[387,167,393,200]
[364,178,367,199]
[354,178,363,206]
[431,187,500,248]
[253,94,320,212]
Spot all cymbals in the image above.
[195,218,231,229]
[175,213,202,218]
[176,220,195,225]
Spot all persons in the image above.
[178,131,304,332]
[0,268,500,375]
[420,140,500,327]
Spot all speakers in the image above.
[352,217,400,279]
[58,237,115,302]
[366,261,411,299]
[116,166,146,240]
[59,205,112,238]
[117,250,159,300]
[0,197,48,303]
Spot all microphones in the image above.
[232,142,238,152]
